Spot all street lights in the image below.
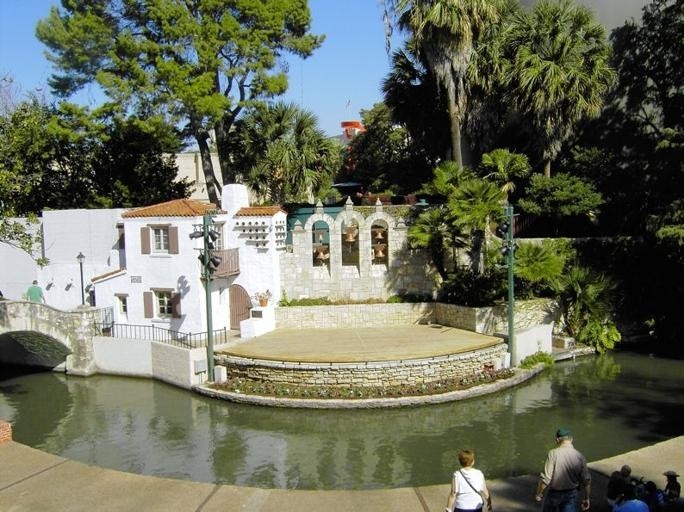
[189,215,223,382]
[75,252,86,305]
[496,206,520,365]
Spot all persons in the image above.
[24,279,45,327]
[443,448,493,512]
[643,481,666,511]
[612,484,649,512]
[534,425,591,511]
[607,465,633,508]
[0,291,11,329]
[663,470,681,503]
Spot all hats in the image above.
[555,427,572,438]
[662,469,681,477]
